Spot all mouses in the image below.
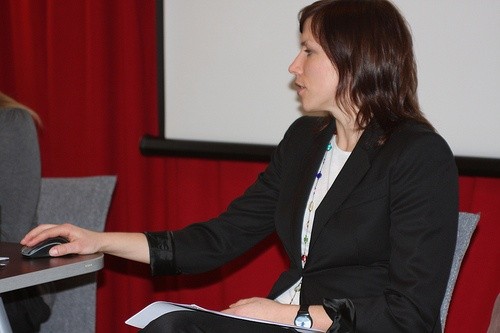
[21,236,70,258]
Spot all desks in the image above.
[0,242,104,333]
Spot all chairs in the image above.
[35,175,117,333]
[440,212,480,333]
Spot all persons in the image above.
[20,0,458,333]
[1,77,52,333]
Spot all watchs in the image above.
[293,303,314,330]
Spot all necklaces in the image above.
[284,142,333,308]
